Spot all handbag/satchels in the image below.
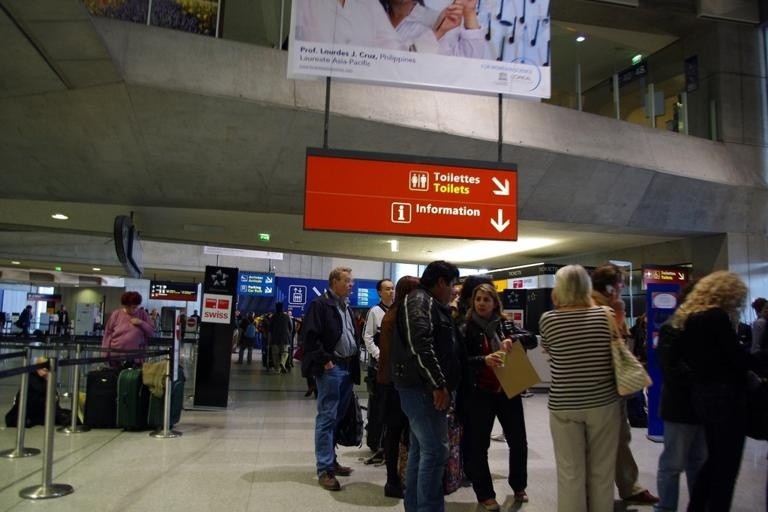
[396,420,466,496]
[599,305,654,396]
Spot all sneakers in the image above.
[621,489,661,505]
[514,489,529,503]
[480,498,499,511]
[304,385,315,398]
[280,361,286,374]
[646,434,665,443]
[317,463,353,491]
[490,434,508,442]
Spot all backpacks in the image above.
[245,318,256,338]
[333,389,369,450]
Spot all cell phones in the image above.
[605,284,616,294]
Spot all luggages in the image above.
[80,364,185,431]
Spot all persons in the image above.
[361,261,537,511]
[56,305,69,335]
[303,0,486,58]
[297,265,361,490]
[19,305,33,334]
[191,303,297,375]
[99,291,157,369]
[539,260,768,511]
